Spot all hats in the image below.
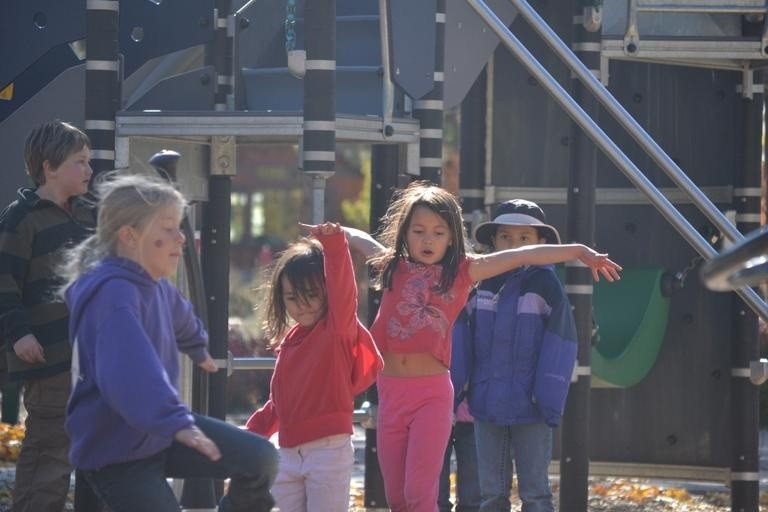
[475,198,561,246]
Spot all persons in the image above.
[59,175,280,512]
[437,424,481,512]
[243,219,384,511]
[1,120,97,512]
[449,197,580,512]
[300,184,622,512]
